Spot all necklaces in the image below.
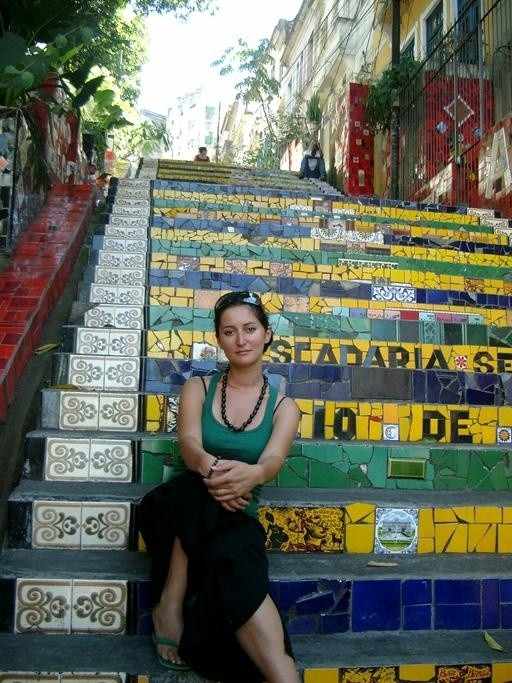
[219,365,269,433]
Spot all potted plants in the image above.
[304,90,322,133]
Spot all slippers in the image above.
[150,627,192,672]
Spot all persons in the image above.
[193,147,210,161]
[134,290,306,683]
[298,141,328,180]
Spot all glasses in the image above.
[214,290,261,312]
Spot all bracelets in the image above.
[206,455,223,480]
